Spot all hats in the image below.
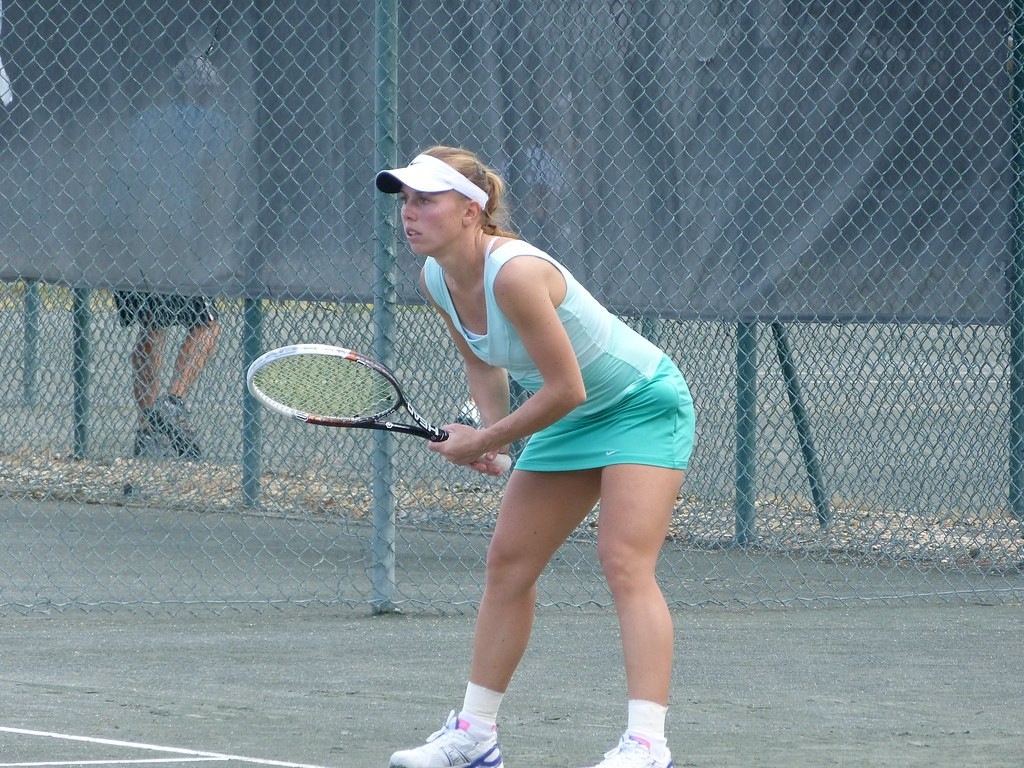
[171,56,221,87]
[374,153,490,211]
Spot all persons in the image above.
[112,288,219,462]
[374,145,695,768]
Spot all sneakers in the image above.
[148,392,202,460]
[134,430,178,457]
[388,708,504,768]
[588,734,673,768]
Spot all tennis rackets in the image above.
[247,344,512,473]
